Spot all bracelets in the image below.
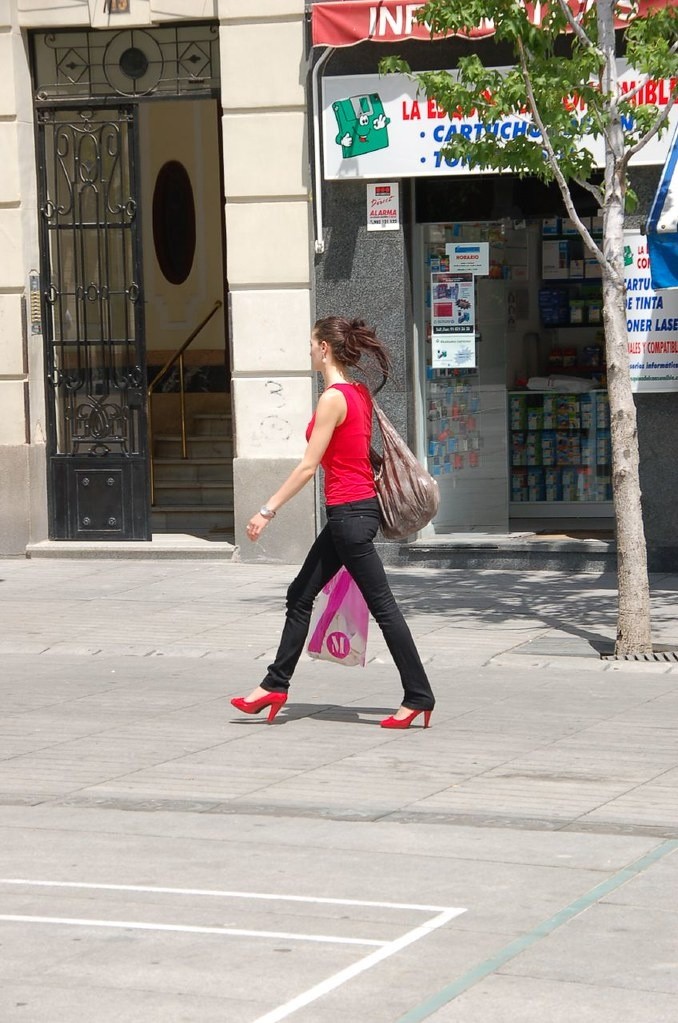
[260,506,276,518]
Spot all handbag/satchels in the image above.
[304,566,368,667]
[370,392,440,545]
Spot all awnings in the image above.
[647,124,678,290]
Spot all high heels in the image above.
[230,692,288,724]
[381,709,432,729]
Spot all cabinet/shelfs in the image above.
[410,221,536,534]
[506,389,617,532]
[539,217,606,379]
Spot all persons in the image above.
[231,317,434,729]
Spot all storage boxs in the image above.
[582,237,601,258]
[570,259,584,278]
[542,239,570,280]
[562,217,602,236]
[585,260,601,278]
[541,218,559,235]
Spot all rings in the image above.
[246,526,250,529]
